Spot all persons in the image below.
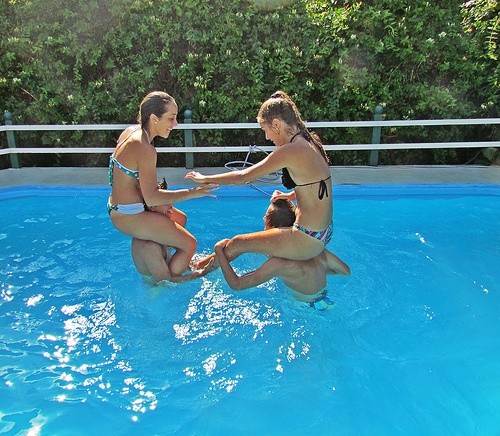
[215,197,351,314]
[184,90,334,272]
[130,179,217,286]
[106,91,221,275]
